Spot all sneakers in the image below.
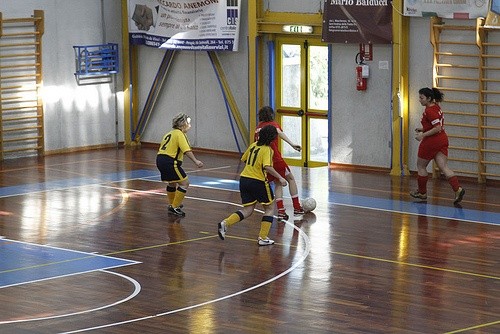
[278,212,289,220]
[218,222,226,240]
[179,204,183,208]
[294,208,306,214]
[168,205,186,216]
[258,236,275,246]
[409,190,427,199]
[453,186,465,204]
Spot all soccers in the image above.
[302,196,316,211]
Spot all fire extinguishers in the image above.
[355,58,367,91]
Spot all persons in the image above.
[410,87,466,205]
[254,106,305,219]
[156,113,203,217]
[218,125,287,246]
